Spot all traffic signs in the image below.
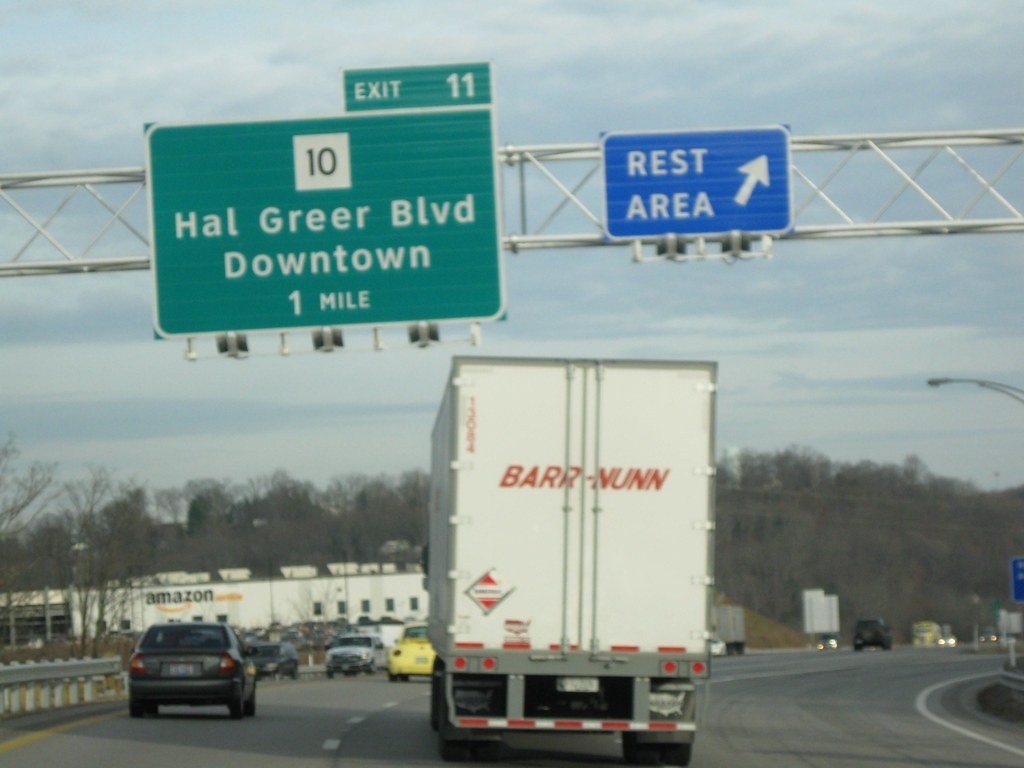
[141,104,508,344]
[598,124,795,241]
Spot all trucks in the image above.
[413,349,719,768]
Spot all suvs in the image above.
[323,633,388,679]
[388,622,439,684]
[128,620,260,720]
[243,641,300,682]
[852,616,894,651]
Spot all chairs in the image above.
[161,631,181,645]
[204,638,221,646]
